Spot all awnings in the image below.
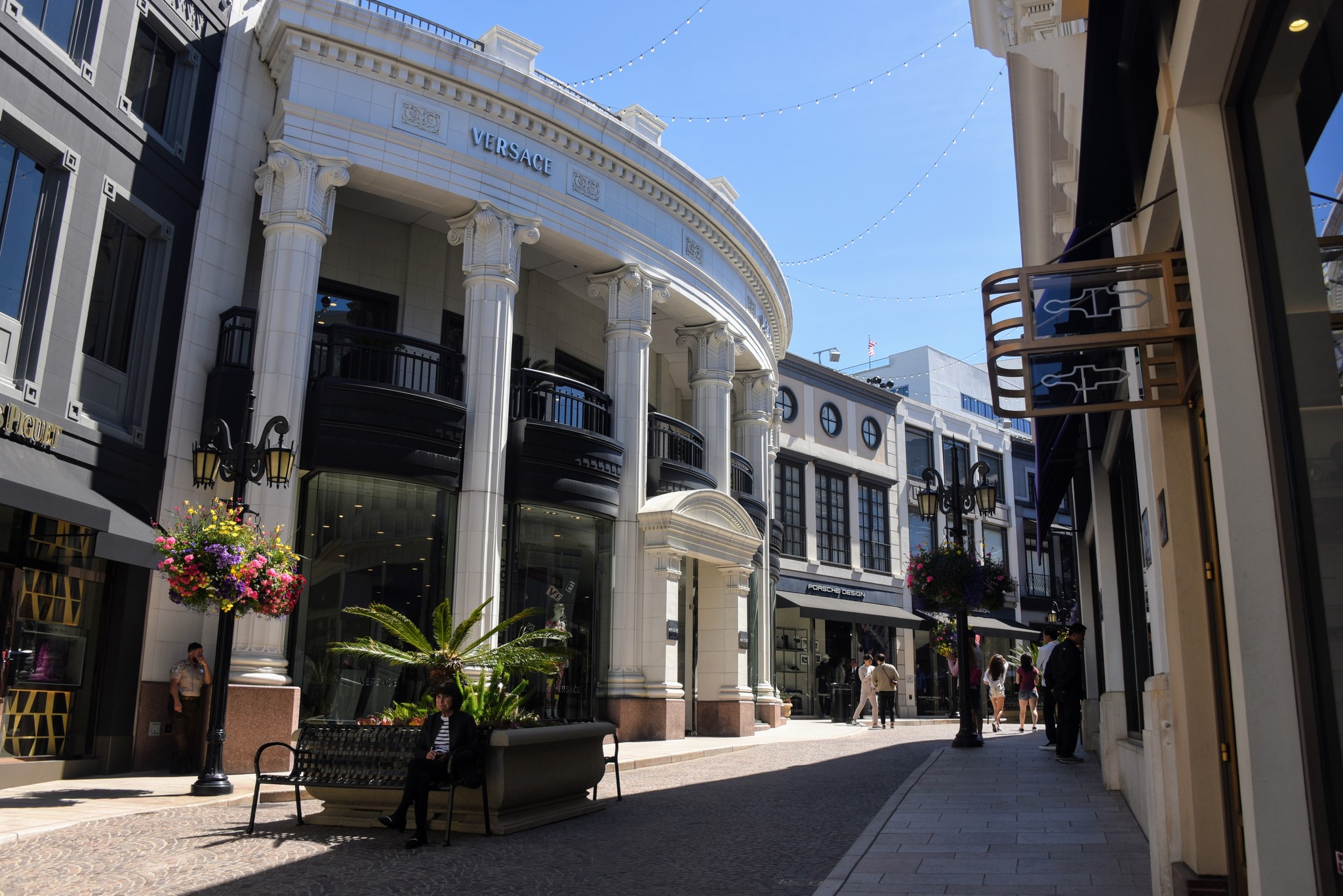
[0,433,111,532]
[915,609,1041,641]
[776,590,921,630]
[1034,384,1083,566]
[94,499,172,571]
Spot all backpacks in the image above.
[969,664,981,685]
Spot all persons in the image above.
[983,654,1008,732]
[835,655,846,694]
[1043,622,1087,763]
[1036,626,1060,748]
[847,658,864,719]
[915,660,926,696]
[161,642,213,776]
[858,623,881,667]
[816,654,835,715]
[1016,652,1039,733]
[378,682,482,849]
[945,630,984,744]
[542,604,569,719]
[852,654,899,728]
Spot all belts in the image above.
[182,695,198,700]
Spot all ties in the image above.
[853,668,855,673]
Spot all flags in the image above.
[868,339,877,356]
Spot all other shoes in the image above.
[378,815,406,833]
[997,728,1002,731]
[171,762,194,775]
[882,725,887,729]
[405,832,427,850]
[872,724,882,728]
[852,720,856,725]
[978,735,985,744]
[781,665,801,671]
[992,721,997,732]
[890,723,894,728]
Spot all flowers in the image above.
[150,495,308,619]
[1056,630,1069,643]
[932,618,974,660]
[780,689,793,704]
[901,528,1018,619]
[1070,603,1077,625]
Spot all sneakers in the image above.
[1059,755,1084,763]
[1039,742,1057,750]
[1032,727,1037,732]
[1018,727,1024,733]
[1055,755,1061,759]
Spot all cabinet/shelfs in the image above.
[775,625,808,715]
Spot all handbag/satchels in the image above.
[891,680,897,686]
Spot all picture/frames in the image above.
[816,654,821,664]
[800,654,807,665]
[815,641,819,651]
[801,637,807,651]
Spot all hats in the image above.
[822,654,831,659]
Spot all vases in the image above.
[781,703,793,718]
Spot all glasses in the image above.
[841,660,846,662]
[851,662,856,664]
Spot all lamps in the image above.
[813,347,841,365]
[330,300,337,306]
[880,381,894,388]
[867,376,882,385]
[992,418,1011,428]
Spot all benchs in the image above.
[246,723,497,847]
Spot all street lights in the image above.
[916,434,1000,747]
[189,414,299,797]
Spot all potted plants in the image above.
[292,595,620,836]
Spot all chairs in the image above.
[593,734,622,802]
